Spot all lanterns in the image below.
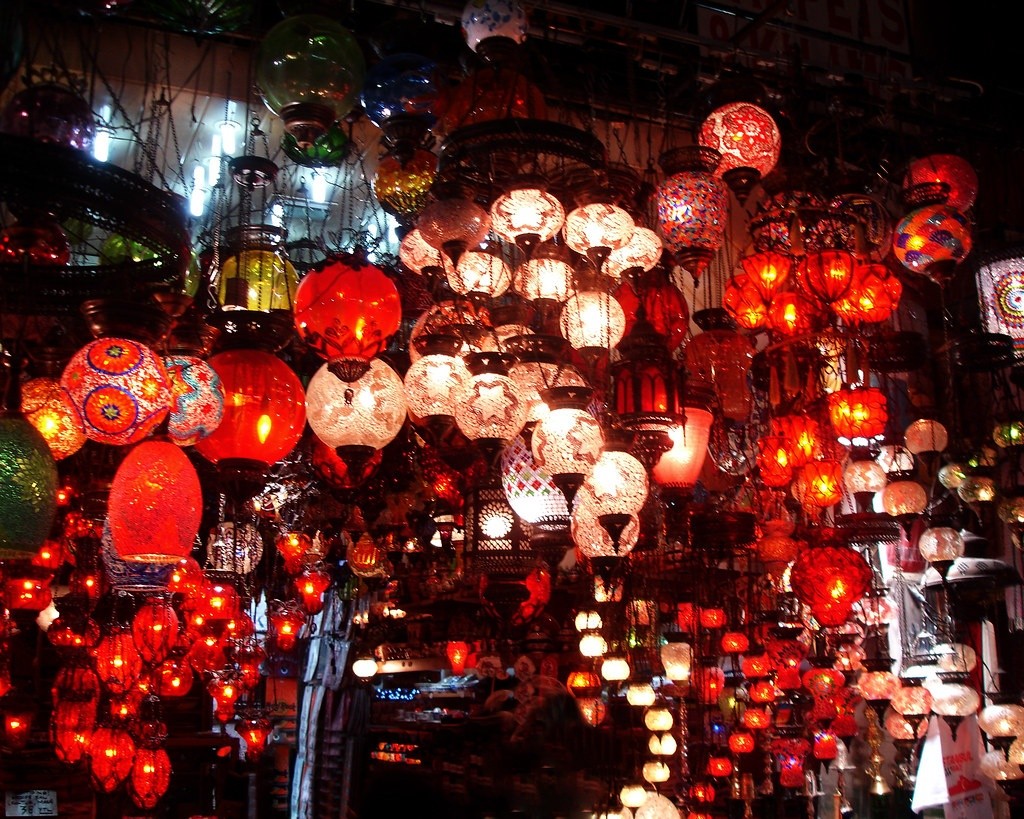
[0,0,1024,819]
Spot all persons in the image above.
[469,657,528,819]
[536,691,584,819]
[513,655,540,819]
